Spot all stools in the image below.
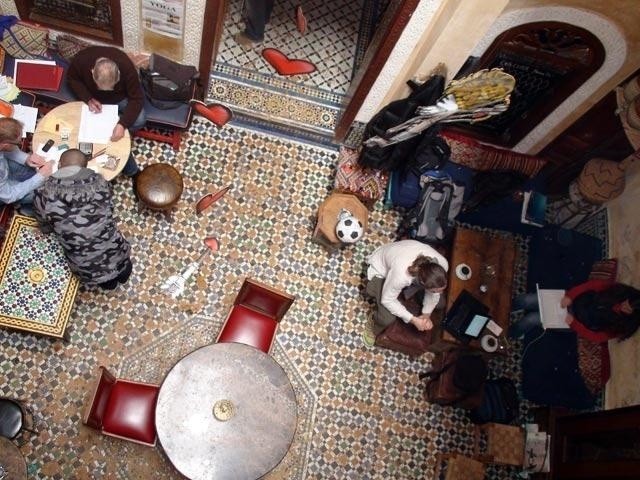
[474,423,524,467]
[427,351,482,410]
[434,452,486,480]
[376,301,436,357]
[312,194,368,252]
[1,399,41,444]
[336,145,382,205]
[136,163,184,218]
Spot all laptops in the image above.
[444,303,492,339]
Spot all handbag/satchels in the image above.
[140,65,195,111]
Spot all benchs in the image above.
[10,52,194,147]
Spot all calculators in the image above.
[79,142,93,160]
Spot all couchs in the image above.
[386,130,618,408]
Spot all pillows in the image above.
[56,35,89,60]
[1,20,50,62]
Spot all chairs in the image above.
[216,278,295,356]
[82,366,159,449]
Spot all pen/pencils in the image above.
[89,97,100,110]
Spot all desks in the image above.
[1,214,80,342]
[0,434,28,480]
[155,342,297,480]
[33,101,130,183]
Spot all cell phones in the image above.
[42,139,55,153]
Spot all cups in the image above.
[460,265,470,276]
[486,337,497,347]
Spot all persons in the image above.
[0,117,55,217]
[363,242,449,349]
[234,1,274,48]
[34,149,132,291]
[65,46,147,177]
[508,279,640,343]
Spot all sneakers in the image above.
[234,31,252,45]
[362,313,376,347]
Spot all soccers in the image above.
[335,216,363,244]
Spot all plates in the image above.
[455,263,473,281]
[480,334,499,353]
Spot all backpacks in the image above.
[413,136,452,178]
[400,179,465,244]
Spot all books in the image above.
[521,191,548,228]
[16,62,63,94]
